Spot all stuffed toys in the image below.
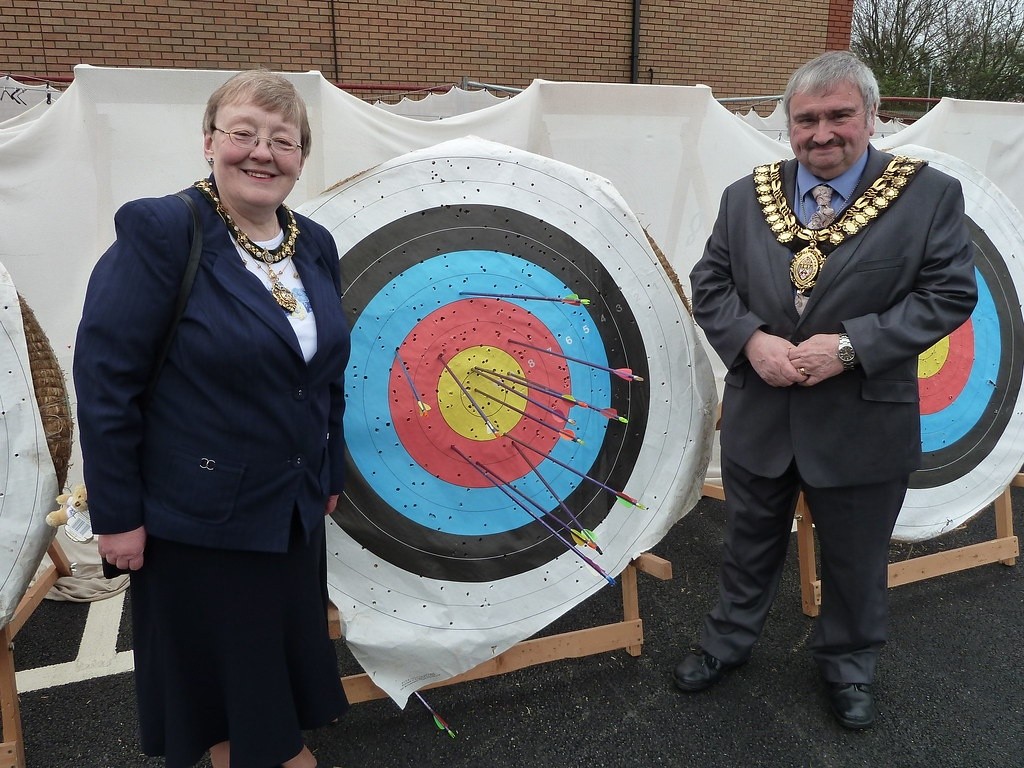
[45,483,93,544]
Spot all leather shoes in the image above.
[826,681,876,728]
[672,647,728,692]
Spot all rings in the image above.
[799,368,805,376]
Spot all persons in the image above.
[73,69,351,768]
[670,51,979,728]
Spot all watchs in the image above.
[835,333,856,372]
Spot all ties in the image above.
[796,185,834,320]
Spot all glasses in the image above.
[212,126,303,155]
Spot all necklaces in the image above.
[193,179,309,320]
[753,154,929,296]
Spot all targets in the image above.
[886,146,1024,544]
[0,261,73,637]
[281,136,697,631]
